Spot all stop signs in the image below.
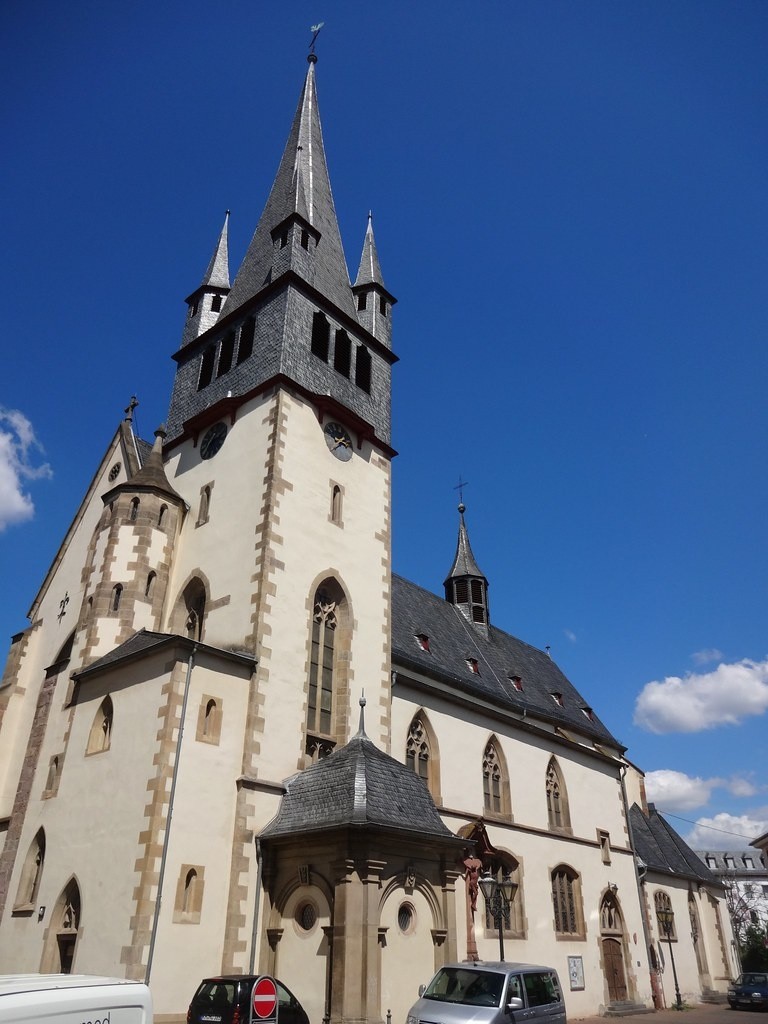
[253,980,278,1018]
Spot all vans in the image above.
[405,962,567,1024]
[1,975,154,1024]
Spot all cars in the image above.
[187,975,311,1024]
[726,973,768,1010]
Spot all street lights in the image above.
[478,872,518,961]
[657,908,686,1010]
